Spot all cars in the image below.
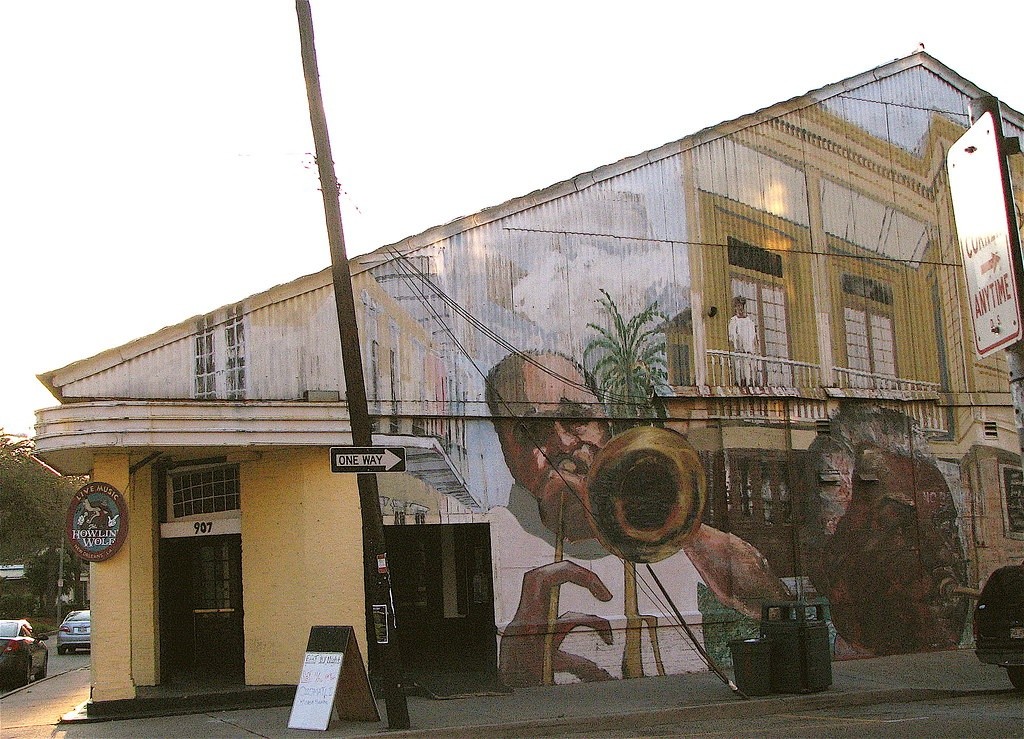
[972,559,1024,690]
[0,619,49,689]
[56,610,91,653]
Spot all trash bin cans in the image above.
[726,636,773,698]
[761,599,834,696]
